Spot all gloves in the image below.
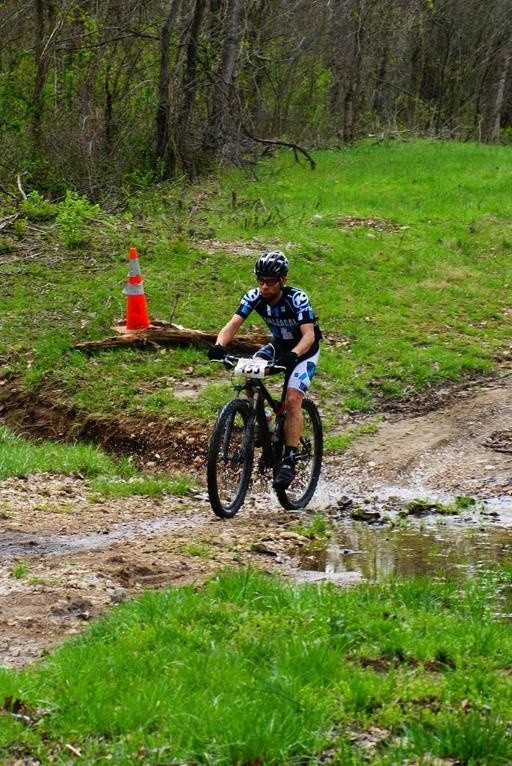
[207,344,227,361]
[269,351,298,374]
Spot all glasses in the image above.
[256,277,281,286]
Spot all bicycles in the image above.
[207,345,323,519]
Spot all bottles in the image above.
[264,404,273,433]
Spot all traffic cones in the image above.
[109,246,161,334]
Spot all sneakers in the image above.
[272,464,297,487]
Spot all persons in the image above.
[206,250,322,492]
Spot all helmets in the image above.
[254,251,290,277]
